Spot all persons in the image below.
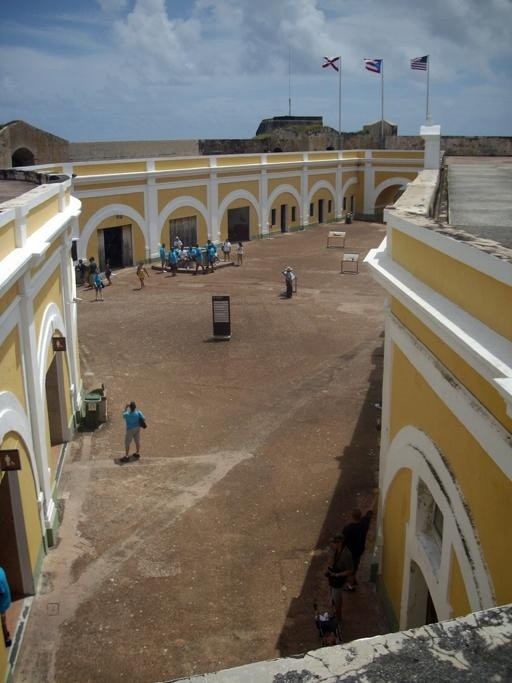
[345,209,352,222]
[118,401,146,462]
[332,487,380,592]
[0,566,13,648]
[76,236,244,301]
[282,267,295,300]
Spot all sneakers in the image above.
[120,455,130,461]
[285,292,292,297]
[159,260,243,276]
[80,280,115,301]
[133,452,140,457]
[140,285,145,289]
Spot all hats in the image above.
[284,266,294,271]
[129,399,136,408]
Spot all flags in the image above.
[411,56,427,71]
[364,57,382,74]
[320,55,341,72]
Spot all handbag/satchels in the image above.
[137,271,138,275]
[140,418,147,429]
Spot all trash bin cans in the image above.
[84,391,107,431]
[346,213,352,224]
